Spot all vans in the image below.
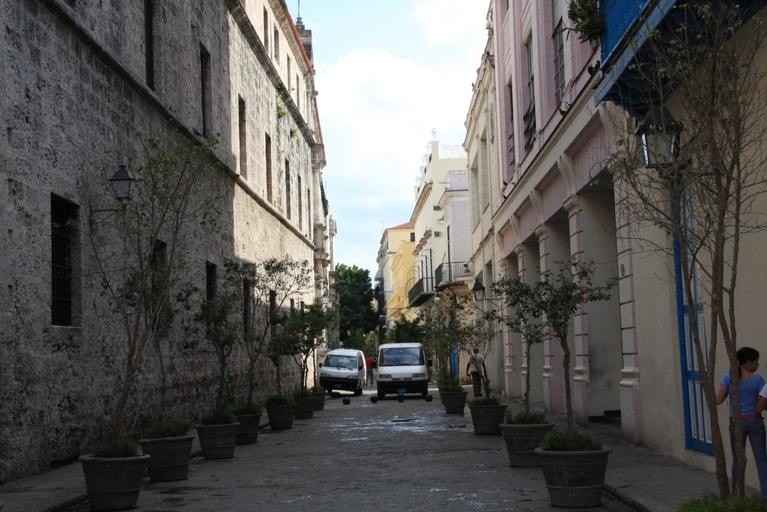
[319,348,367,397]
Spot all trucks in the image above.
[372,343,433,400]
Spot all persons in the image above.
[755,378,767,415]
[715,346,767,501]
[366,354,375,387]
[466,347,488,397]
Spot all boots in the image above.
[474,393,483,397]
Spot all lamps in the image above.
[632,97,684,169]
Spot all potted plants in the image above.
[423,289,467,413]
[483,276,555,466]
[78,124,237,511]
[227,257,315,445]
[179,270,240,459]
[457,295,507,436]
[307,298,325,411]
[520,254,626,508]
[136,224,192,483]
[266,305,336,419]
